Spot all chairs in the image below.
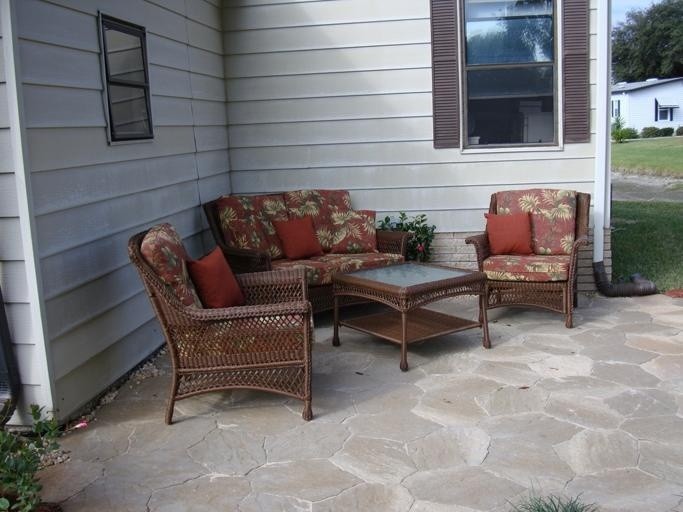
[127,221,314,422]
[464,188,590,328]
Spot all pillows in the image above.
[186,245,245,310]
[270,208,380,262]
[485,211,535,258]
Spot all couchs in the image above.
[200,186,413,316]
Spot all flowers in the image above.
[377,214,435,261]
